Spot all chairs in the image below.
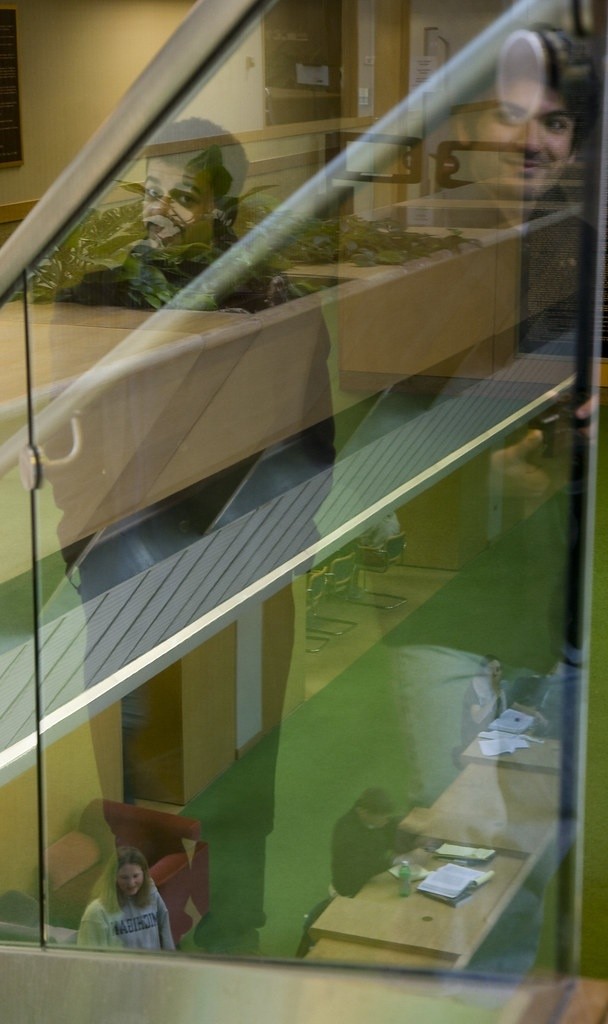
[306,551,358,636]
[334,530,409,610]
[306,566,331,653]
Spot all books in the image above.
[388,843,496,904]
[476,708,544,756]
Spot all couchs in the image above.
[24,797,210,946]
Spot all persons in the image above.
[461,654,545,743]
[345,512,401,599]
[340,21,600,955]
[331,788,395,899]
[41,118,336,955]
[77,846,176,951]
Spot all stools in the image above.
[294,898,335,960]
[452,745,470,770]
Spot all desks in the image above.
[302,655,565,971]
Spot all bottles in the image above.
[398,861,411,896]
